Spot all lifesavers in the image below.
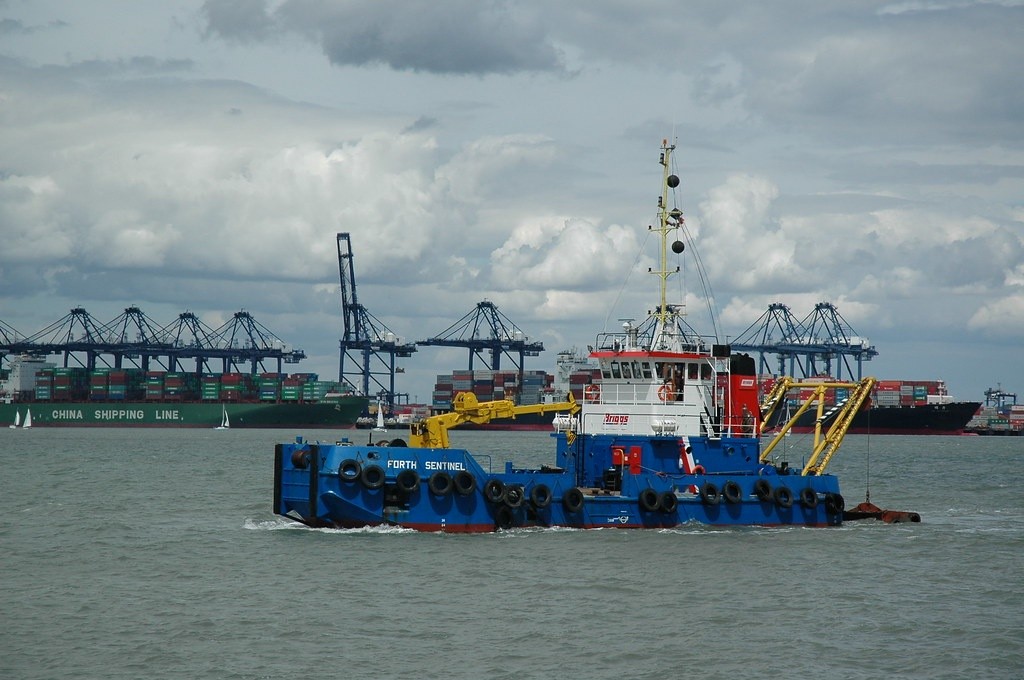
[657,385,672,401]
[692,465,706,475]
[585,384,600,400]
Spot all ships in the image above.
[0,355,368,429]
[382,367,1022,437]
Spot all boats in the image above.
[270,135,875,532]
